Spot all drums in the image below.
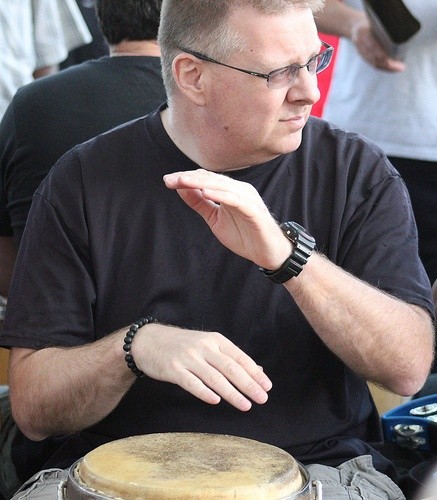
[55,431,324,500]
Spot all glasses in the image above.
[175,37,334,89]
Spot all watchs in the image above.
[257,220,315,284]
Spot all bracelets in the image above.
[124,316,162,378]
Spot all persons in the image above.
[0,0,167,321]
[0,0,94,123]
[311,0,437,286]
[0,0,437,500]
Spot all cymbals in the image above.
[380,392,437,451]
